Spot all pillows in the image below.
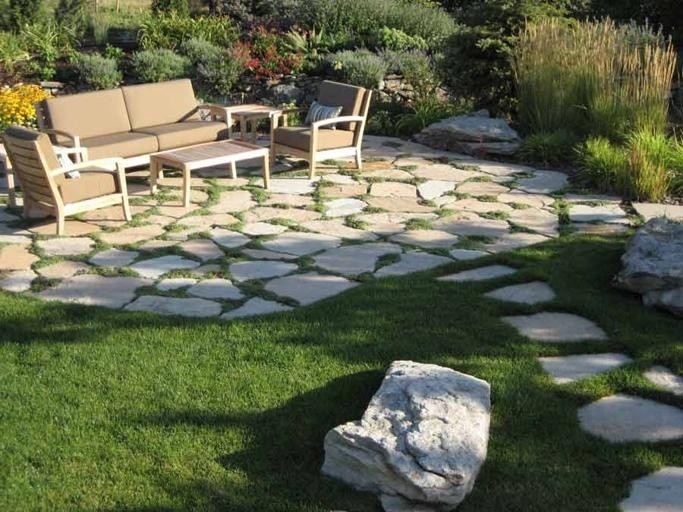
[53,144,82,180]
[303,99,343,131]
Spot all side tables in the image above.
[223,103,281,142]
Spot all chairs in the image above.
[272,78,373,180]
[2,124,133,237]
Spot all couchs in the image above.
[36,77,238,179]
